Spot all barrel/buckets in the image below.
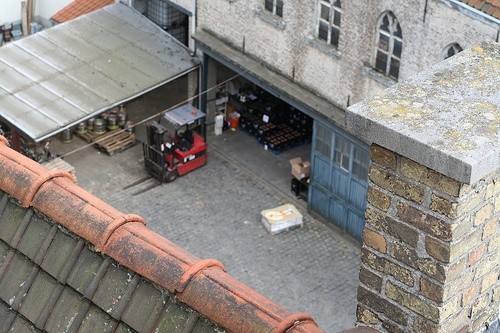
[61,107,135,143]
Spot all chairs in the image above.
[173,130,196,150]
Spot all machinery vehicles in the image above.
[145,104,210,180]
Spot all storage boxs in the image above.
[289,155,311,179]
[258,202,304,234]
